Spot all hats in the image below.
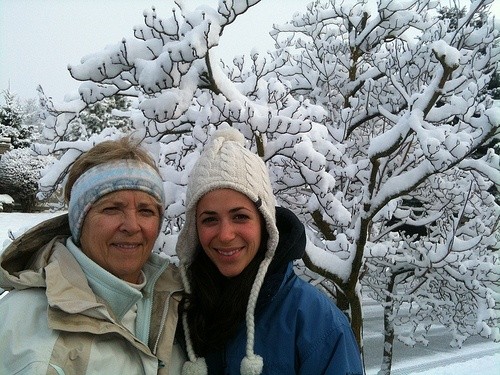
[174,121,280,375]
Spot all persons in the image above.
[173,127,364,375]
[0,129,188,375]
